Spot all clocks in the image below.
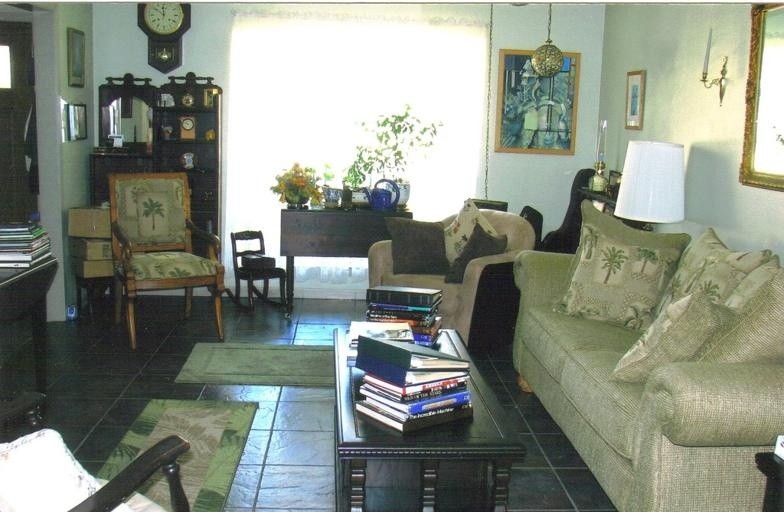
[137,3,191,74]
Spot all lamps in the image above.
[533,4,563,77]
[614,142,686,233]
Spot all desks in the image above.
[1,260,59,424]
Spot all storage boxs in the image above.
[66,206,112,237]
[71,260,114,279]
[69,238,115,260]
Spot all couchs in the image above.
[369,212,535,351]
[514,247,784,511]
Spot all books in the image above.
[0,221,52,267]
[346,320,414,367]
[366,285,443,348]
[354,336,473,432]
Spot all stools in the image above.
[76,277,113,318]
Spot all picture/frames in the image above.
[67,28,84,86]
[624,70,645,131]
[495,49,580,155]
[67,103,87,142]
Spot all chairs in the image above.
[109,173,224,349]
[231,230,286,311]
[2,392,190,512]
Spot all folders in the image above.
[357,336,470,370]
[365,285,443,308]
[355,354,470,387]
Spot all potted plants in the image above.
[343,162,372,205]
[272,164,318,209]
[374,104,435,207]
[322,165,341,207]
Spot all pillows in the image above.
[443,199,500,265]
[651,228,771,326]
[724,255,776,311]
[690,269,783,363]
[385,217,448,275]
[613,284,731,386]
[444,224,508,286]
[553,202,689,336]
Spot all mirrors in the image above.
[738,3,783,194]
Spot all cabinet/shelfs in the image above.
[91,75,221,271]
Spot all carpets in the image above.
[97,396,259,512]
[174,342,336,389]
